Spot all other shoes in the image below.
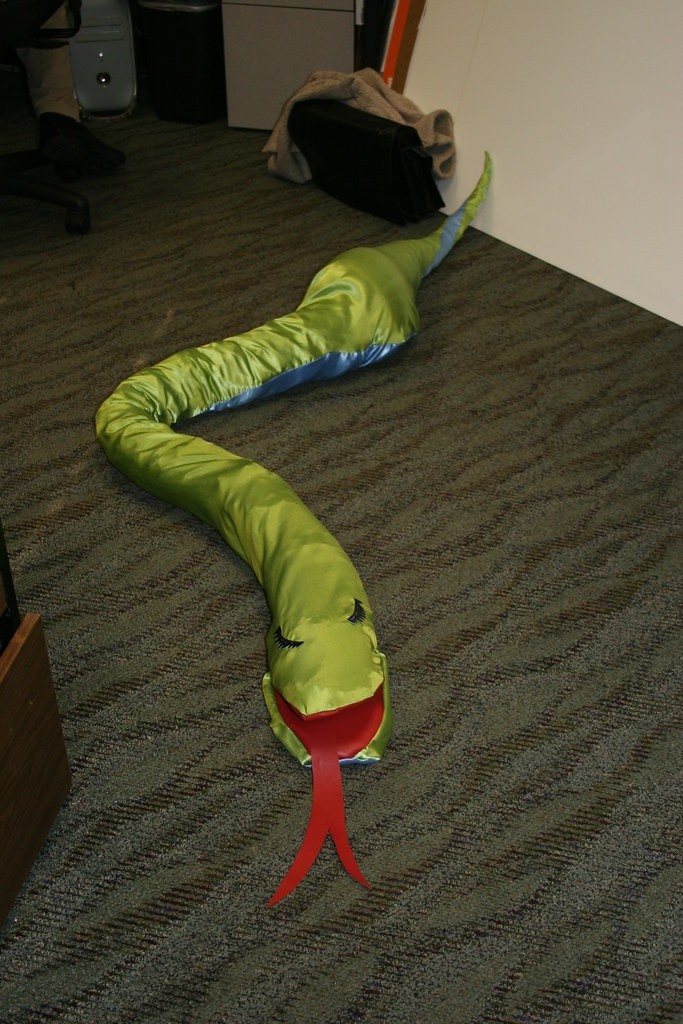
[37,110,128,168]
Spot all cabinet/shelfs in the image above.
[0,527,72,925]
[221,0,357,130]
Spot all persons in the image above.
[14,6,126,175]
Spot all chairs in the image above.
[0,0,128,233]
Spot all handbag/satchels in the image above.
[286,96,443,226]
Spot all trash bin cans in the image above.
[136,0,222,126]
